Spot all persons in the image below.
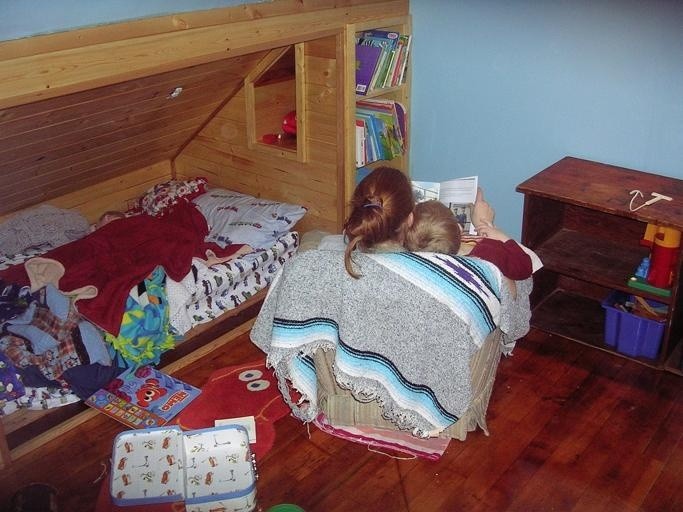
[344,168,517,300]
[407,200,533,282]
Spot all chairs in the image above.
[276,253,527,462]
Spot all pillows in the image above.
[185,188,305,257]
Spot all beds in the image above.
[0,182,346,503]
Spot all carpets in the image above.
[100,355,302,512]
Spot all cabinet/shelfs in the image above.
[515,155,682,394]
[343,12,413,223]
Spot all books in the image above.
[356,99,409,168]
[82,362,203,431]
[409,175,478,245]
[355,28,413,95]
